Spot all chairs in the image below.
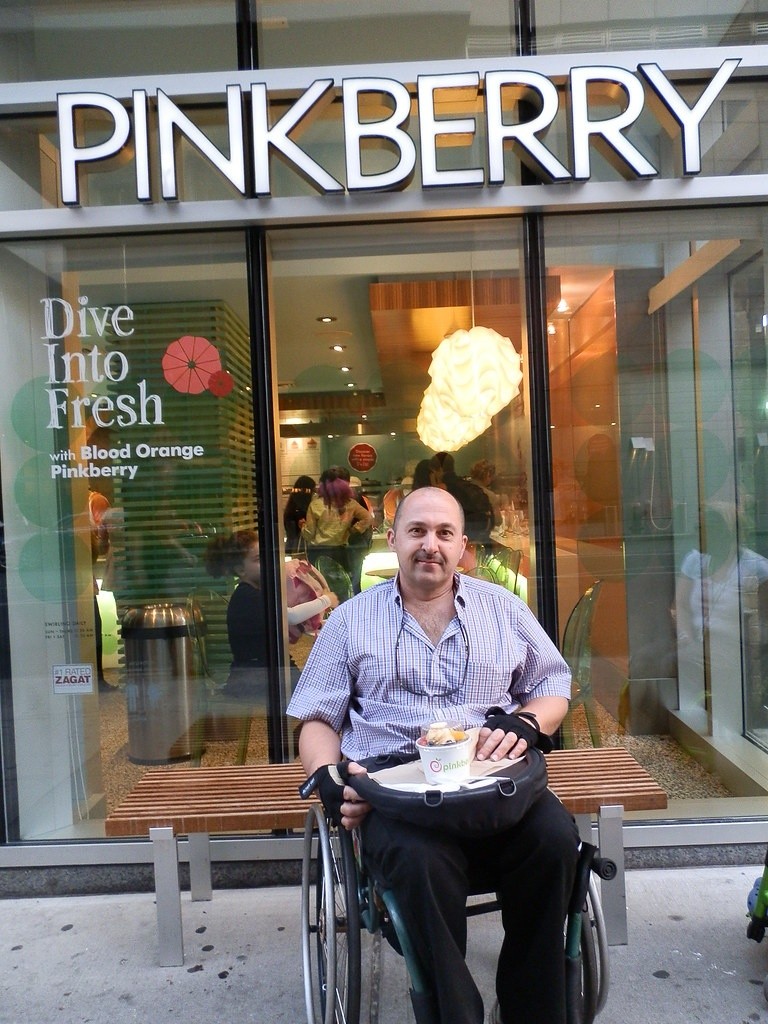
[185,584,267,766]
[316,555,356,605]
[465,546,521,596]
[558,577,610,750]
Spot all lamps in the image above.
[415,252,521,456]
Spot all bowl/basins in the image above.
[415,733,473,786]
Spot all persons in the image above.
[206,529,339,760]
[287,486,572,1023]
[284,452,503,596]
[676,500,768,730]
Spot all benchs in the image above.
[104,747,670,967]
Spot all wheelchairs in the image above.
[298,764,618,1024]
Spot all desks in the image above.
[361,554,527,615]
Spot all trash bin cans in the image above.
[117,600,212,768]
